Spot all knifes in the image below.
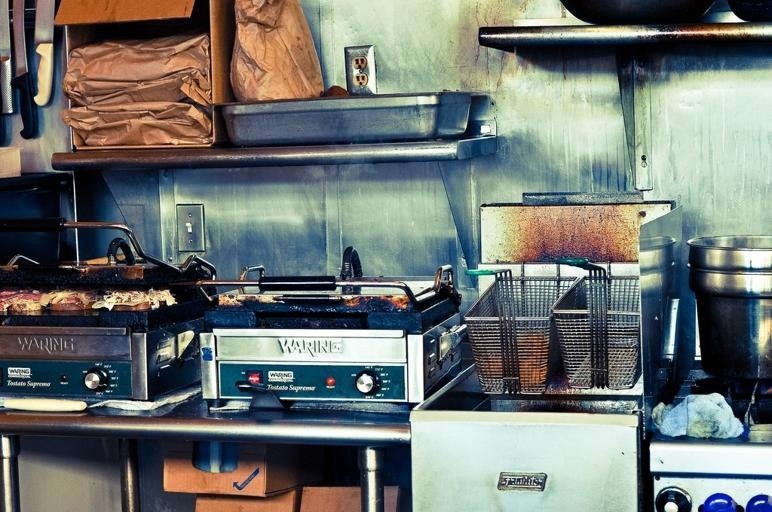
[13,0,35,139]
[0,0,12,114]
[33,0,56,107]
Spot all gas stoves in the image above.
[650,370,772,511]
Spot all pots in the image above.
[686,235,772,380]
[640,235,675,390]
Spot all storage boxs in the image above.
[195,491,294,511]
[54,0,234,149]
[163,439,299,496]
[300,487,405,512]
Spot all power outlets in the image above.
[346,46,375,96]
[177,203,207,252]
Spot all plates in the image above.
[210,90,480,144]
